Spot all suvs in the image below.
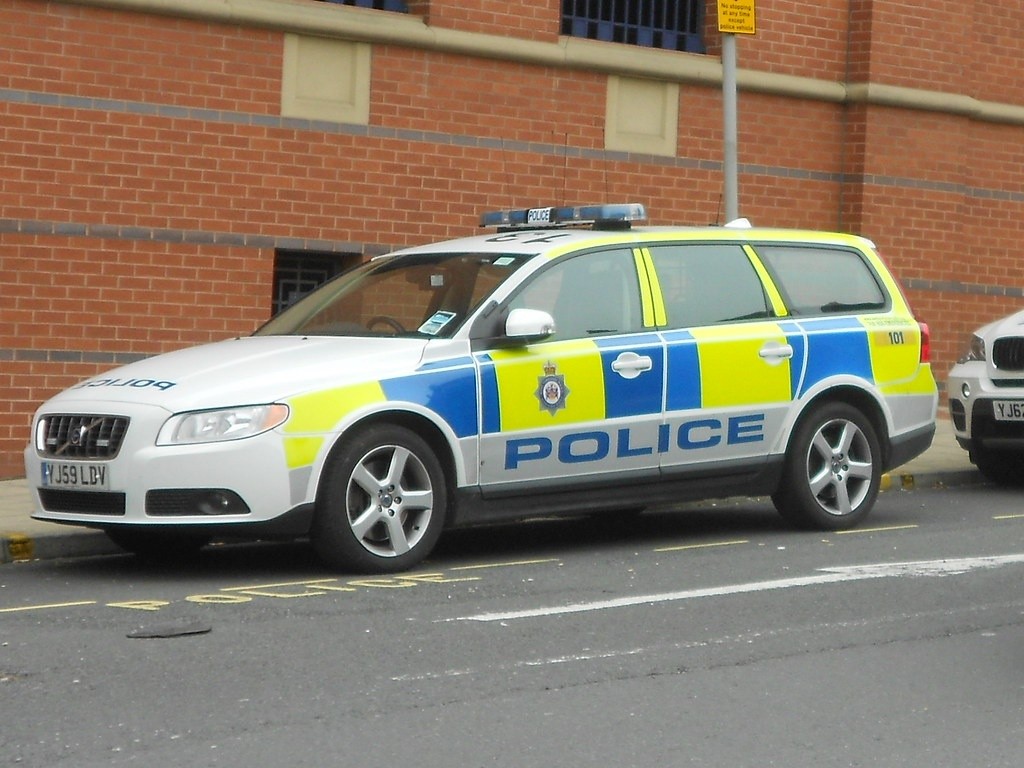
[947,308,1024,486]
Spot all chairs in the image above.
[556,273,616,342]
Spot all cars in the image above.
[23,202,940,574]
